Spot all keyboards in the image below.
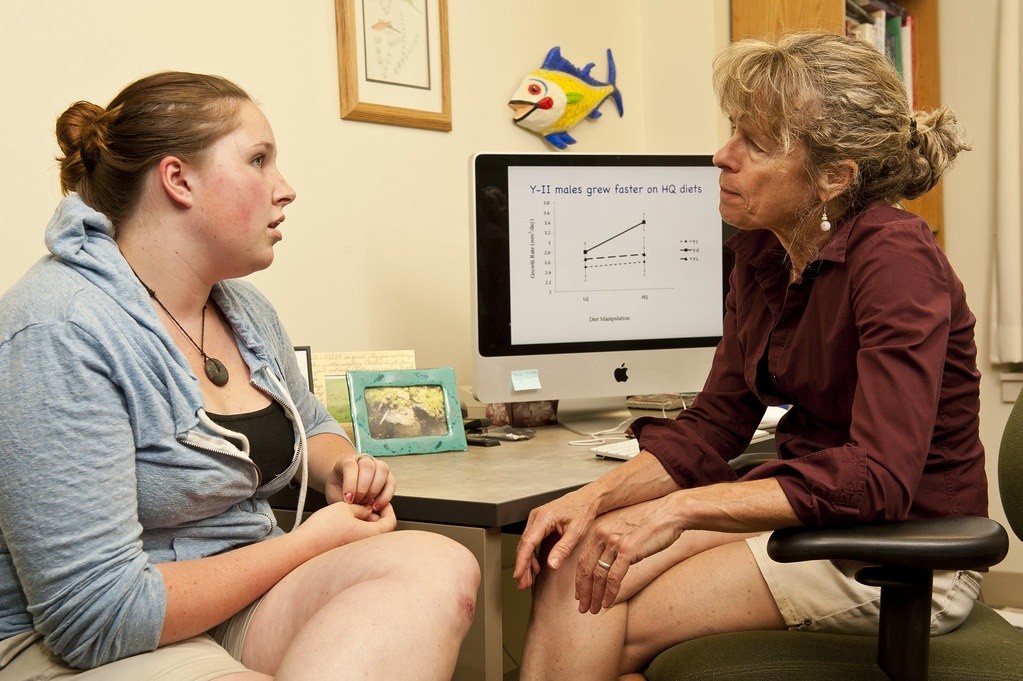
[591,429,770,461]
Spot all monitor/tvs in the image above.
[467,152,735,436]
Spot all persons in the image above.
[1,73,480,681]
[513,34,990,681]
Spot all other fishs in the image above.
[508,46,624,150]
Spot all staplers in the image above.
[463,419,501,447]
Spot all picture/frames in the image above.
[346,365,468,456]
[334,0,453,133]
[293,346,314,394]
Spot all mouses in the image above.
[760,406,790,424]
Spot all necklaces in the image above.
[118,246,229,386]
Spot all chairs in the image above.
[640,385,1023,681]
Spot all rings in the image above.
[598,559,611,570]
[357,455,375,464]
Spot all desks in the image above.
[356,409,780,681]
